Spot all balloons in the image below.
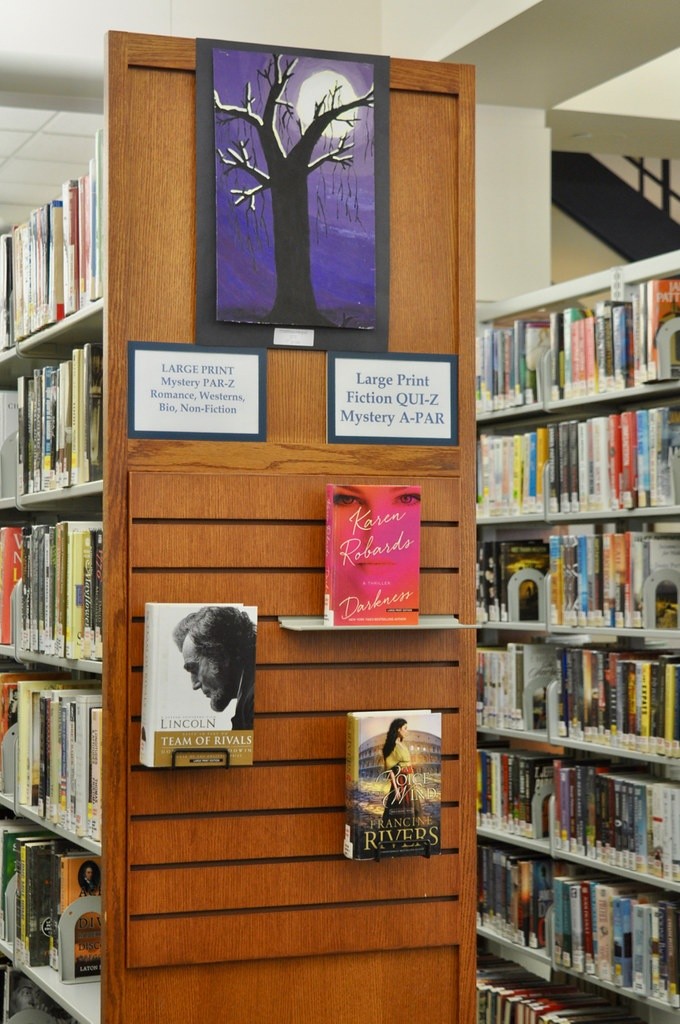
[475,641,680,758]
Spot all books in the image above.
[475,402,680,516]
[1,520,102,663]
[141,603,259,766]
[323,483,421,627]
[2,128,106,353]
[476,276,680,413]
[475,532,679,631]
[475,844,679,1024]
[0,820,102,1024]
[343,709,442,860]
[0,342,103,501]
[476,749,680,882]
[1,668,102,843]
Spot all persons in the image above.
[171,604,254,730]
[383,718,423,828]
[333,486,420,601]
[82,865,95,892]
[11,977,48,1016]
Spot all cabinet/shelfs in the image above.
[0,33,680,1024]
